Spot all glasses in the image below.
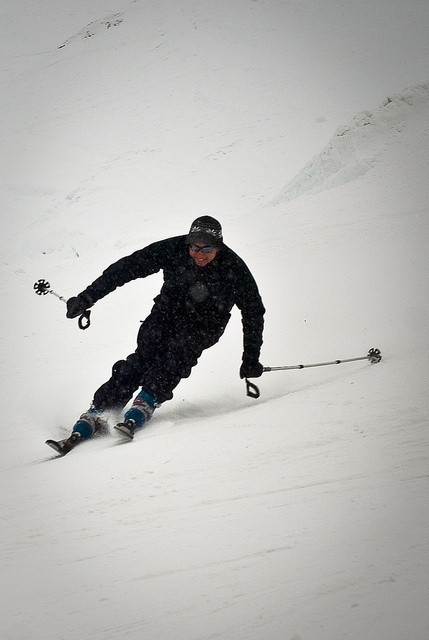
[190,244,213,253]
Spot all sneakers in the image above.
[72,405,101,438]
[125,388,159,426]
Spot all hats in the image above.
[185,216,224,244]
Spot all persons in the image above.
[65,214,266,438]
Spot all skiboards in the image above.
[44,426,134,456]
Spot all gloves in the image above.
[66,290,93,318]
[240,352,263,379]
